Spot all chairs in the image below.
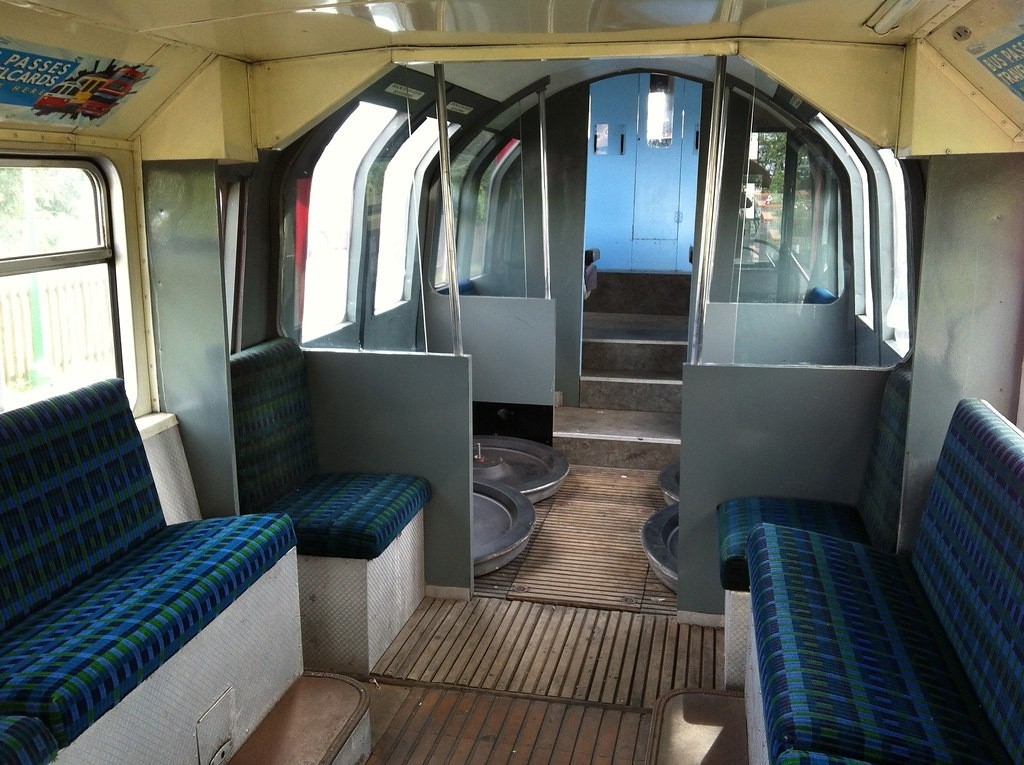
[718,360,916,693]
[581,247,603,302]
[686,243,693,270]
[807,285,840,307]
[452,275,481,296]
[227,333,433,677]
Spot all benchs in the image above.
[0,717,63,764]
[741,394,1022,765]
[776,745,877,763]
[0,374,310,763]
